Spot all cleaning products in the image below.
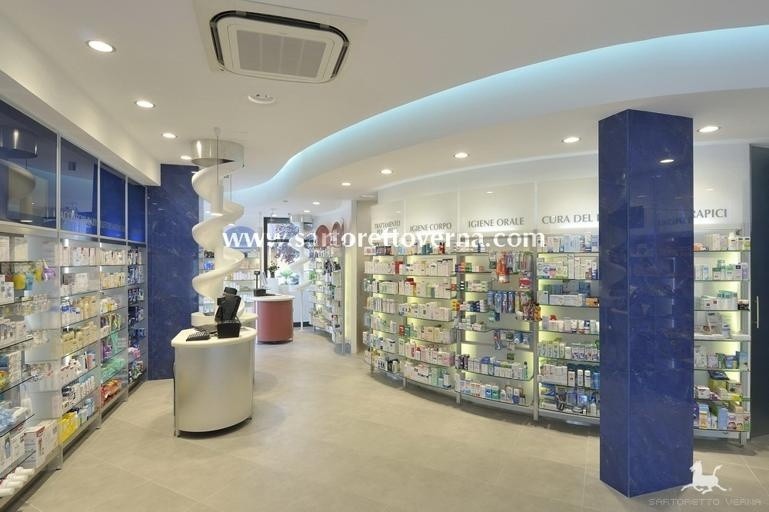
[536,341,599,389]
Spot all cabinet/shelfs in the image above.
[0,219,148,512]
[307,226,751,446]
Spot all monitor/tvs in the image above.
[215,287,237,322]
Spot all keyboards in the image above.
[186,331,210,341]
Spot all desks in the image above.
[171,326,258,437]
